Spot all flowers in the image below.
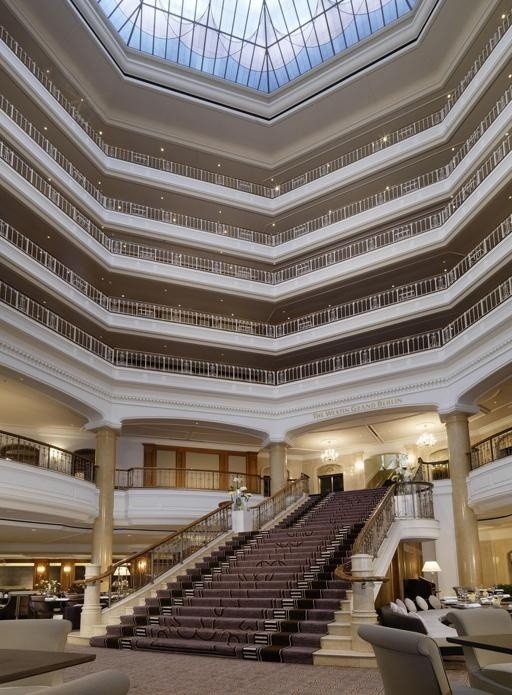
[231,477,252,508]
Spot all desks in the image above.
[0,648,96,684]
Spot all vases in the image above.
[234,494,245,511]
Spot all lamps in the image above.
[416,422,437,448]
[321,439,338,462]
[421,561,441,582]
[113,567,131,594]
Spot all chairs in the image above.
[0,591,129,630]
[31,670,130,695]
[0,619,72,695]
[358,584,512,695]
